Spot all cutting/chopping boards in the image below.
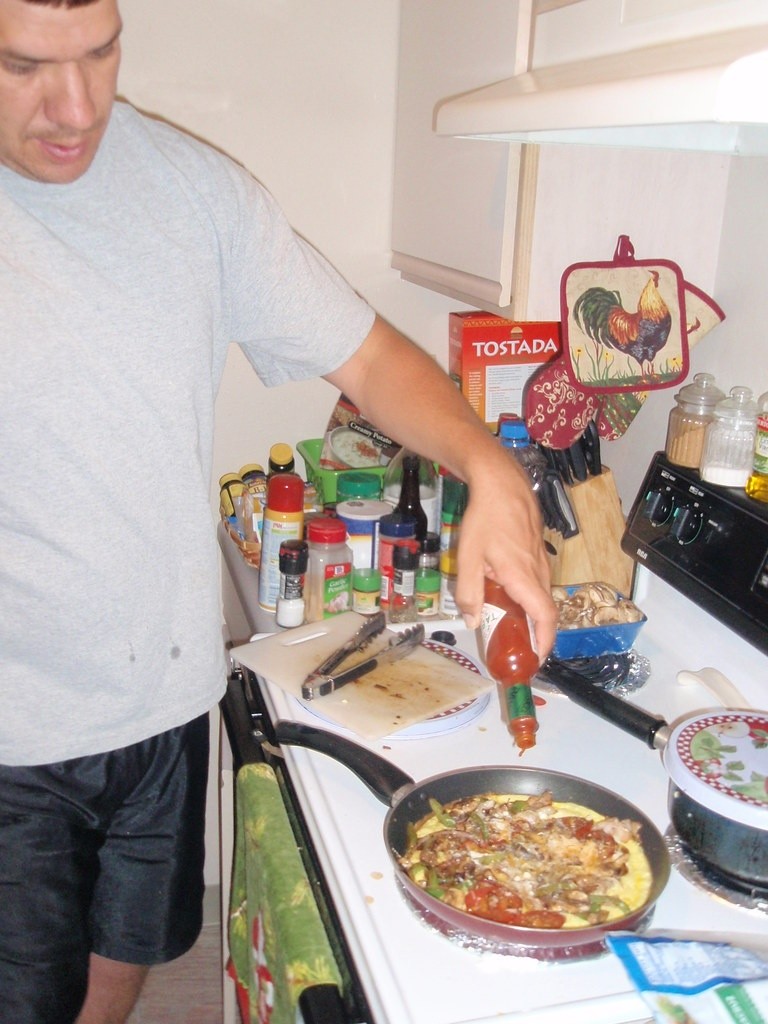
[229,612,494,739]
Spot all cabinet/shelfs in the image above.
[216,523,288,1024]
[388,0,735,323]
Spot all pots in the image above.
[275,721,670,946]
[542,660,768,888]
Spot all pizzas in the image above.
[400,793,652,929]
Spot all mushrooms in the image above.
[550,583,645,630]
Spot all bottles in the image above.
[217,412,528,627]
[482,578,542,749]
[665,372,767,501]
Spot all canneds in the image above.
[334,472,394,570]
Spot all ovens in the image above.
[223,651,347,1024]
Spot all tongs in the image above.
[300,611,425,701]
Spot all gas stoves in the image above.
[245,450,767,1023]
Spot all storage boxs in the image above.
[448,310,564,437]
[548,582,647,660]
[297,439,439,506]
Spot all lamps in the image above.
[434,0,767,156]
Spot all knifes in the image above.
[530,422,602,537]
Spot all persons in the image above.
[1,0,557,1022]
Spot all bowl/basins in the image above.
[548,583,646,659]
[328,426,392,471]
